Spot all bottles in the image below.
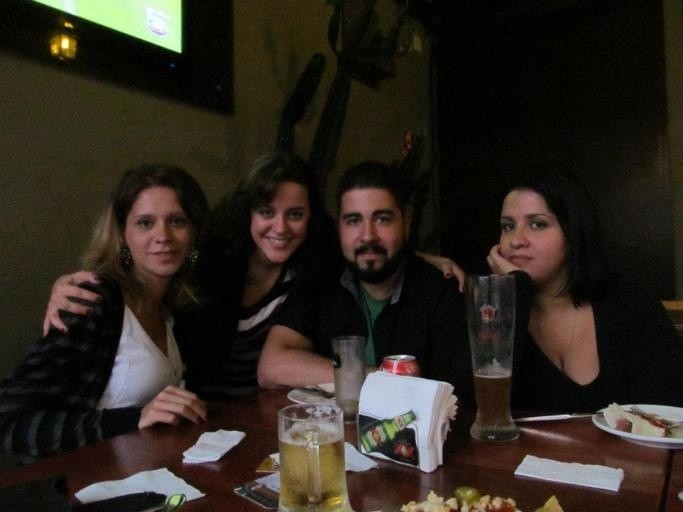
[359,409,416,453]
[378,354,420,380]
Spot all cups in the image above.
[278,402,349,512]
[333,337,365,425]
[467,274,522,444]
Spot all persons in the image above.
[258,160,470,391]
[41,155,474,398]
[1,165,212,462]
[486,173,680,416]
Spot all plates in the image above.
[593,405,683,450]
[285,381,335,406]
